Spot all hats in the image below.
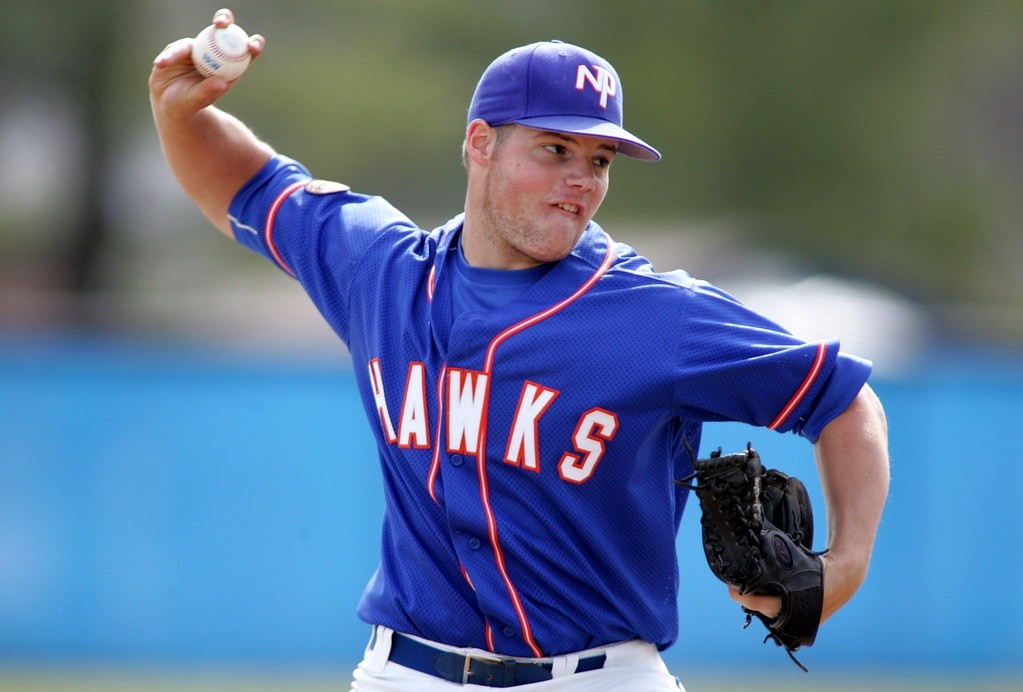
[467,39,661,162]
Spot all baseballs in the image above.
[189,22,253,83]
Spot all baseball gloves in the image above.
[670,436,832,674]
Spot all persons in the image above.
[148,5,891,691]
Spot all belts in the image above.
[370,624,607,688]
